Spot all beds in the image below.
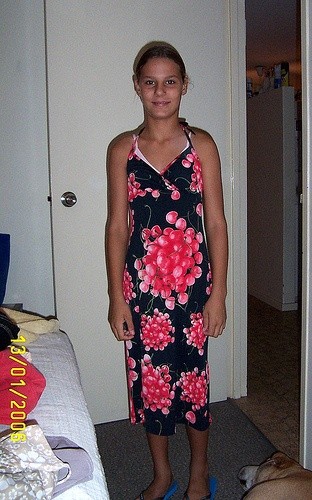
[0,331,110,500]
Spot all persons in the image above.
[104,44,228,500]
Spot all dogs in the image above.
[237,452,312,500]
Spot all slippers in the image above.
[135,480,179,500]
[184,476,217,500]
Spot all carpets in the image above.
[95,401,278,500]
